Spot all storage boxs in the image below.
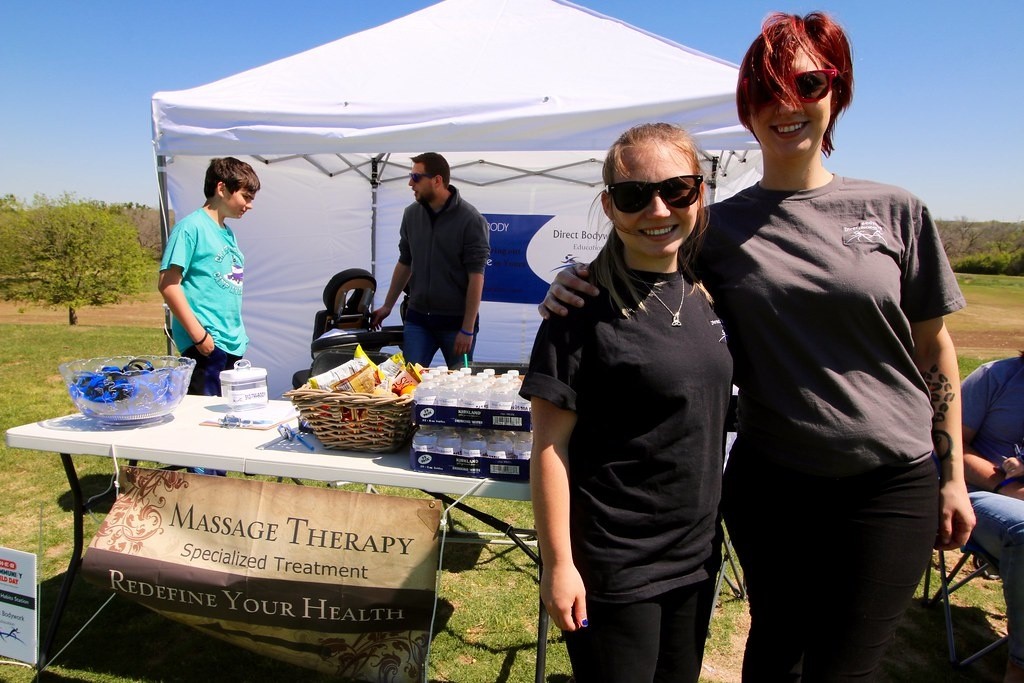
[411,375,533,433]
[409,429,529,485]
[219,360,269,412]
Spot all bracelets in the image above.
[460,328,475,336]
[193,331,208,345]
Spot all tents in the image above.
[152,0,765,406]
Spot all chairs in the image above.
[309,348,394,377]
[705,395,746,635]
[922,452,1008,671]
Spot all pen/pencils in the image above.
[278,423,315,451]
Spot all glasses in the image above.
[409,172,446,183]
[743,69,839,106]
[607,175,704,214]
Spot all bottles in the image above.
[415,366,531,411]
[413,424,532,460]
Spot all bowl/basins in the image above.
[60,356,196,432]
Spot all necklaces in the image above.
[636,273,686,328]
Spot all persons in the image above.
[961,349,1024,683]
[158,158,262,476]
[540,13,977,682]
[369,153,489,371]
[517,122,732,682]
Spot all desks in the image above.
[4,396,549,683]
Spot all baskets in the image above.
[282,374,415,454]
